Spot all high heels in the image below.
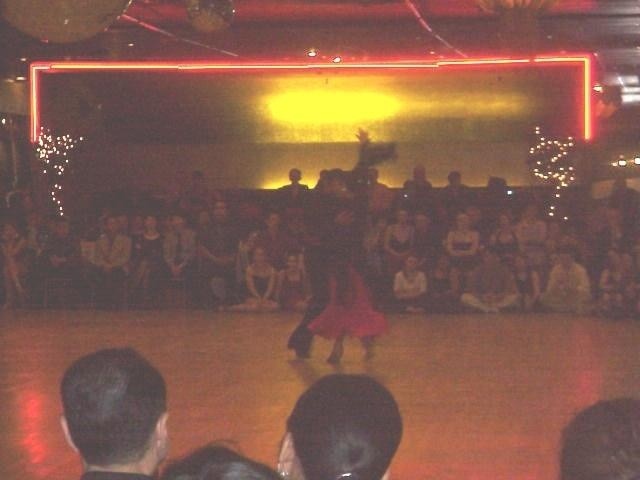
[327,344,343,362]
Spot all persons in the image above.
[278,373,405,480]
[161,445,285,480]
[1,168,310,313]
[59,345,169,480]
[558,399,640,480]
[285,129,399,363]
[382,165,640,313]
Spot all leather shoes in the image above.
[288,340,310,359]
